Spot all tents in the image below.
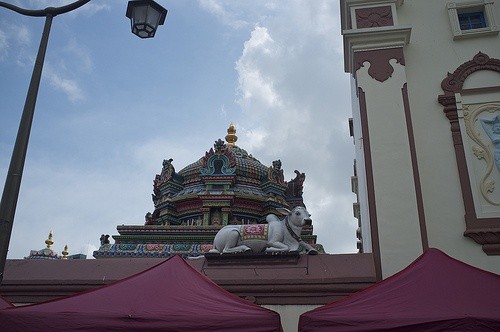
[298,248,500,332]
[2,255,283,332]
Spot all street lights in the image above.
[0,0,166,292]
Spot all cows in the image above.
[186,206,319,260]
